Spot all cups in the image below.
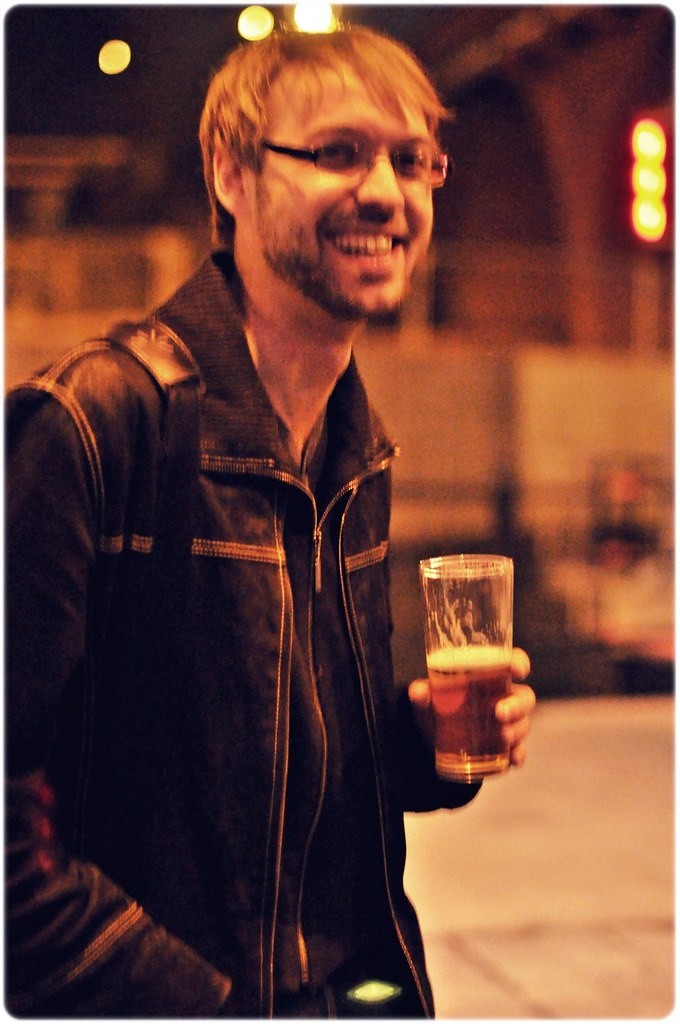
[422,553,516,778]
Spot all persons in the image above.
[5,27,535,1019]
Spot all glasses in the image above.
[257,131,452,188]
